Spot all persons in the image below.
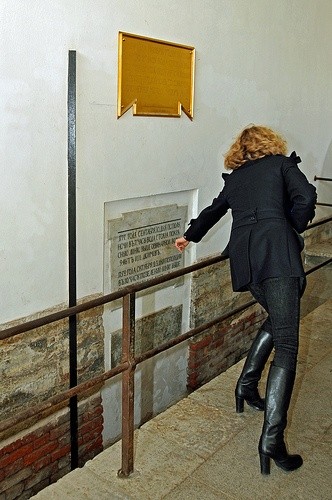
[172,126,319,477]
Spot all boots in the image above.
[258,364,305,477]
[236,332,277,415]
